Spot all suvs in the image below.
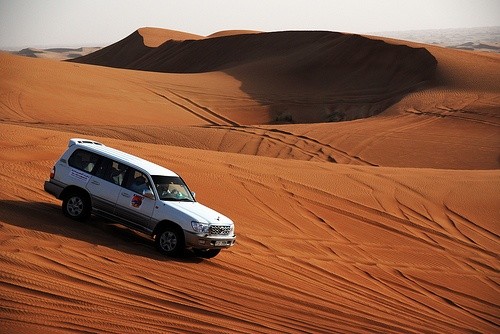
[44,138,238,260]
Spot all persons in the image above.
[157,184,178,197]
